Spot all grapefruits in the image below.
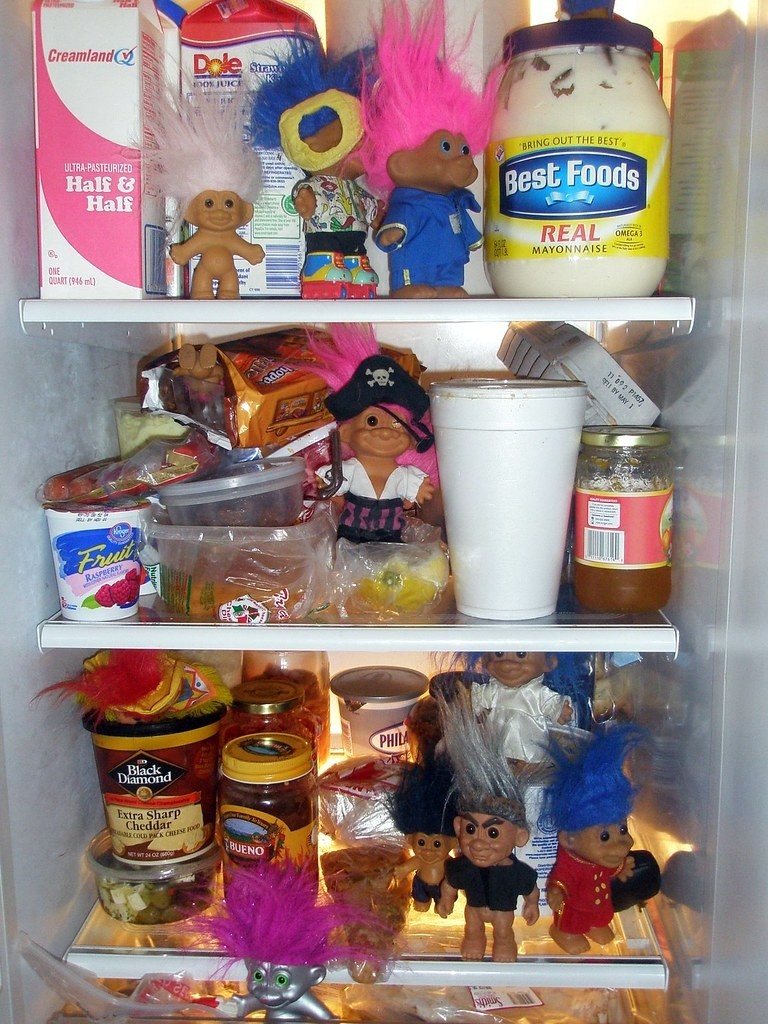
[355,541,450,612]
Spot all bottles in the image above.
[484,20,669,296]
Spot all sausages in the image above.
[43,459,127,501]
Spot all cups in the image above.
[330,665,430,762]
[82,699,226,866]
[429,376,589,621]
[41,503,145,621]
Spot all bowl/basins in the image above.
[158,455,309,525]
[85,826,219,927]
[146,499,333,624]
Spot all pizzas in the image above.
[320,844,414,984]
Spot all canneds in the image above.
[571,424,676,615]
[215,679,321,910]
[482,18,672,296]
